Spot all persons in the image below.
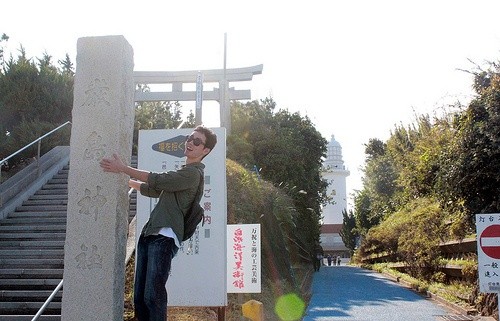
[313,248,341,272]
[100,125,217,321]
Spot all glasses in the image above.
[186,135,209,147]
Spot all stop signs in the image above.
[479,224,500,259]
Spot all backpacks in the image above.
[174,166,206,240]
